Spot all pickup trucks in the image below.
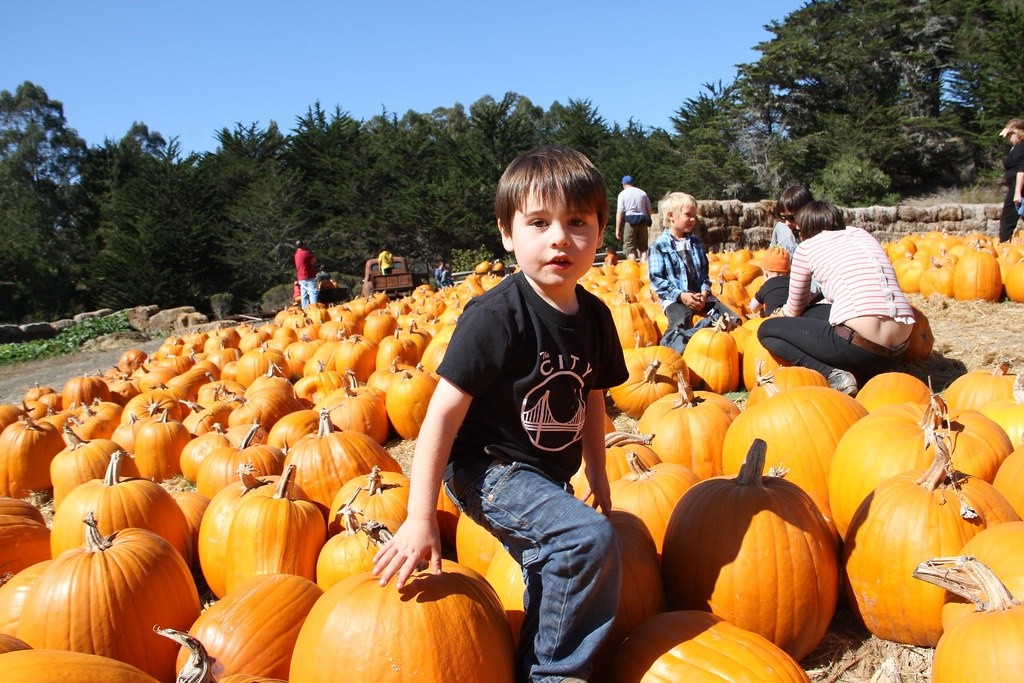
[362,258,430,299]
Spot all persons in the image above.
[378,245,394,275]
[293,265,333,299]
[757,201,916,395]
[615,176,652,263]
[648,192,739,355]
[373,145,630,683]
[435,262,454,290]
[604,245,617,266]
[294,240,318,310]
[749,185,825,319]
[999,119,1024,243]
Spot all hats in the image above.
[999,128,1024,137]
[622,176,634,185]
[762,246,790,273]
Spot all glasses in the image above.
[792,229,800,237]
[778,213,795,223]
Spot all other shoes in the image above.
[827,367,859,396]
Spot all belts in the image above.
[834,324,912,357]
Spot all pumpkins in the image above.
[576,229,1023,681]
[1,258,528,683]
